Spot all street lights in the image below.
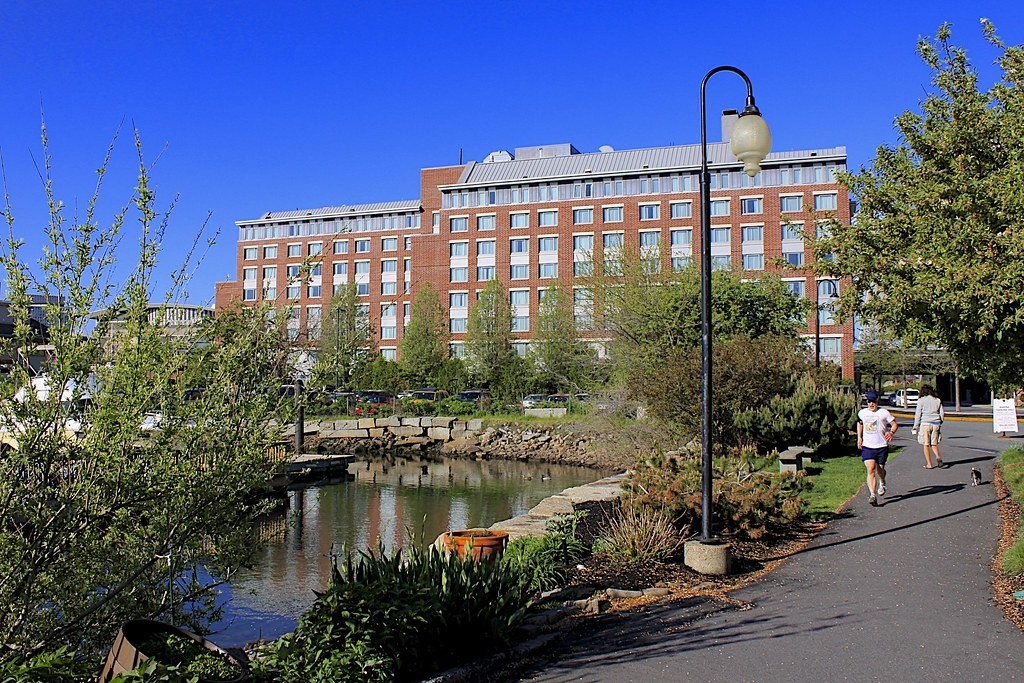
[684,66,773,575]
[336,306,346,391]
[815,279,839,371]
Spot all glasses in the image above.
[868,400,877,403]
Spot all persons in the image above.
[913,384,944,469]
[856,392,899,506]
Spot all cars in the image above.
[889,388,920,407]
[523,394,614,409]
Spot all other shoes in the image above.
[878,481,885,496]
[869,496,877,506]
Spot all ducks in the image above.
[541,474,551,480]
[521,474,533,481]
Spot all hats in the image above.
[867,392,878,400]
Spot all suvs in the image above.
[460,389,493,404]
[270,384,450,409]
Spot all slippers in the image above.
[923,465,933,469]
[938,463,943,468]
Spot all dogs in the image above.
[971,468,981,487]
